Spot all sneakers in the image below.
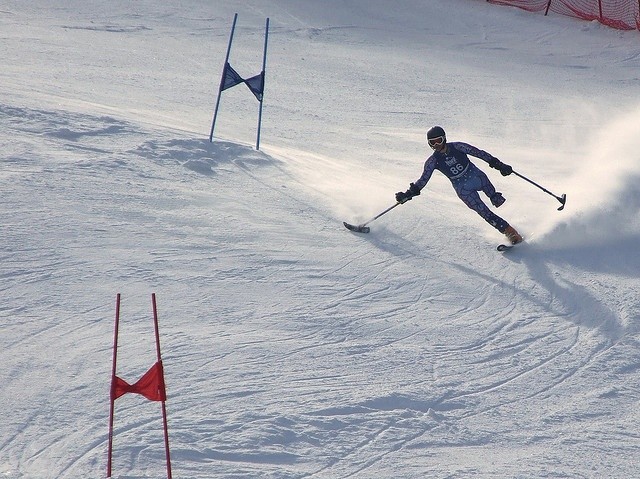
[495,196,505,207]
[501,225,523,243]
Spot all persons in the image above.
[395,126,524,244]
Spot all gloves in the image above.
[496,162,512,176]
[395,190,412,204]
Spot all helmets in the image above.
[427,127,445,138]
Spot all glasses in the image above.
[428,136,445,146]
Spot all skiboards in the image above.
[497,244,515,251]
